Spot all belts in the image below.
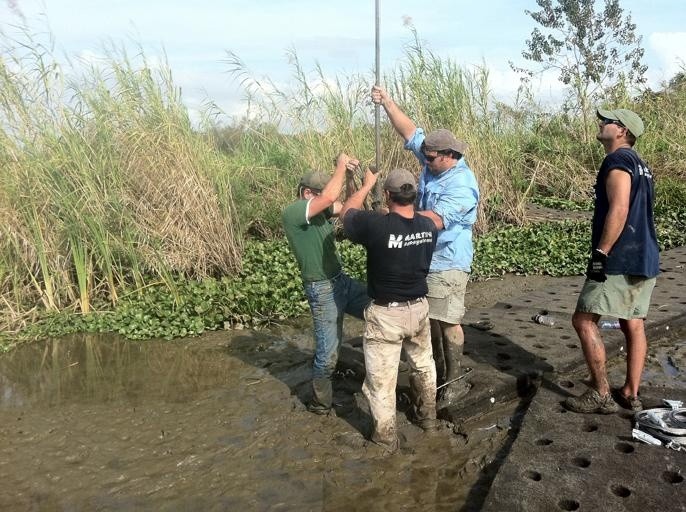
[375,298,420,307]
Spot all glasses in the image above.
[605,119,631,136]
[420,151,441,161]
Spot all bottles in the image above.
[531,314,555,327]
[597,319,621,329]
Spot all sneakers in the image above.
[566,388,619,414]
[610,386,643,412]
[307,402,353,415]
[436,379,472,412]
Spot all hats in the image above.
[384,168,417,193]
[597,109,644,138]
[301,171,332,189]
[425,128,468,154]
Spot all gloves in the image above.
[587,249,608,282]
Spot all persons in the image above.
[370,84,479,413]
[283,154,359,416]
[565,109,660,414]
[340,166,438,454]
